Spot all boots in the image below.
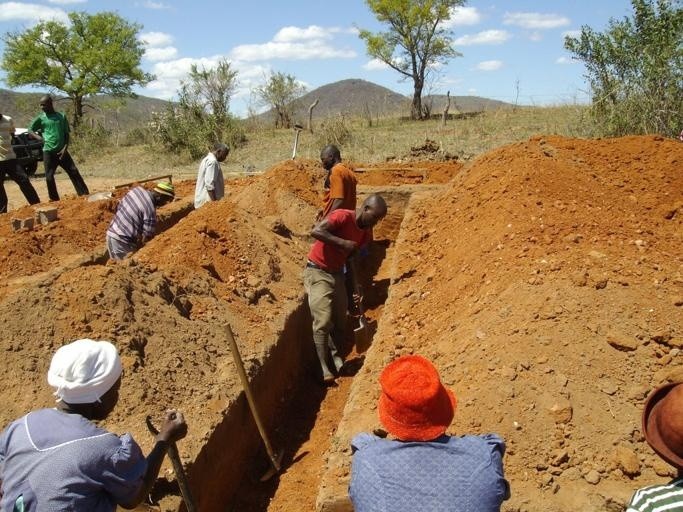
[313,333,334,382]
[328,333,344,376]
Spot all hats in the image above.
[378,355,457,441]
[153,182,175,197]
[642,381,682,468]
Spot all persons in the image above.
[346,358,510,512]
[1,335,187,512]
[302,194,387,382]
[105,181,176,262]
[0,110,41,213]
[193,143,232,212]
[28,94,91,202]
[621,376,682,511]
[314,144,358,311]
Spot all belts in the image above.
[306,262,320,269]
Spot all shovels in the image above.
[340,244,369,354]
